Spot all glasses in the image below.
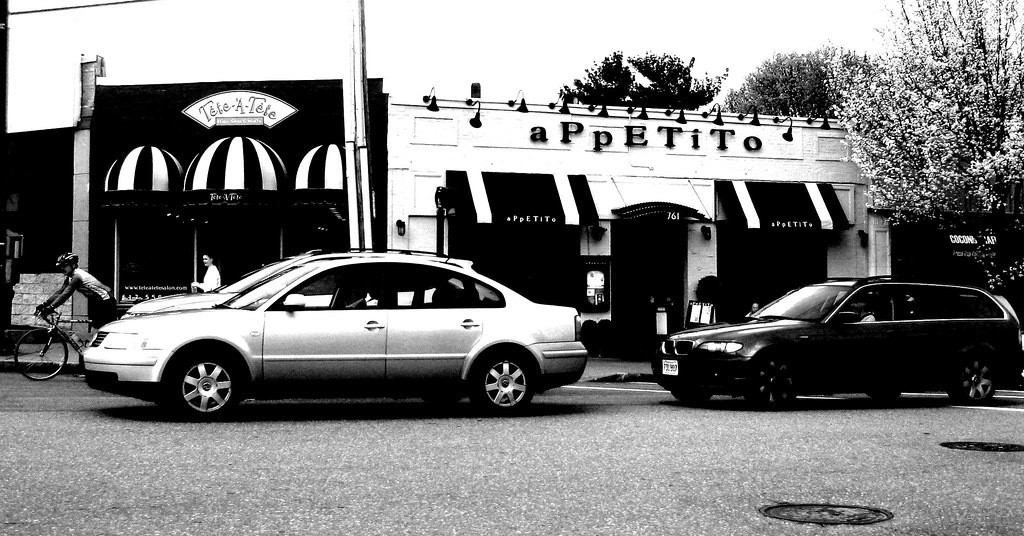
[62,263,69,268]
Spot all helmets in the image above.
[56,253,78,267]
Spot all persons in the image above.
[191,252,221,292]
[851,291,880,322]
[34,253,117,377]
[744,303,759,317]
[342,279,367,308]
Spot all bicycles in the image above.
[15,309,94,382]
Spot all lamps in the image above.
[701,225,711,241]
[859,229,868,248]
[591,226,607,241]
[422,86,833,142]
[396,219,406,236]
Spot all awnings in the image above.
[182,136,293,190]
[446,171,600,226]
[715,181,851,230]
[294,143,348,189]
[105,146,184,192]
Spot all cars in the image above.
[84,259,588,423]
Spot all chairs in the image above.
[353,275,404,308]
[881,296,899,321]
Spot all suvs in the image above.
[121,248,501,320]
[650,275,1024,413]
